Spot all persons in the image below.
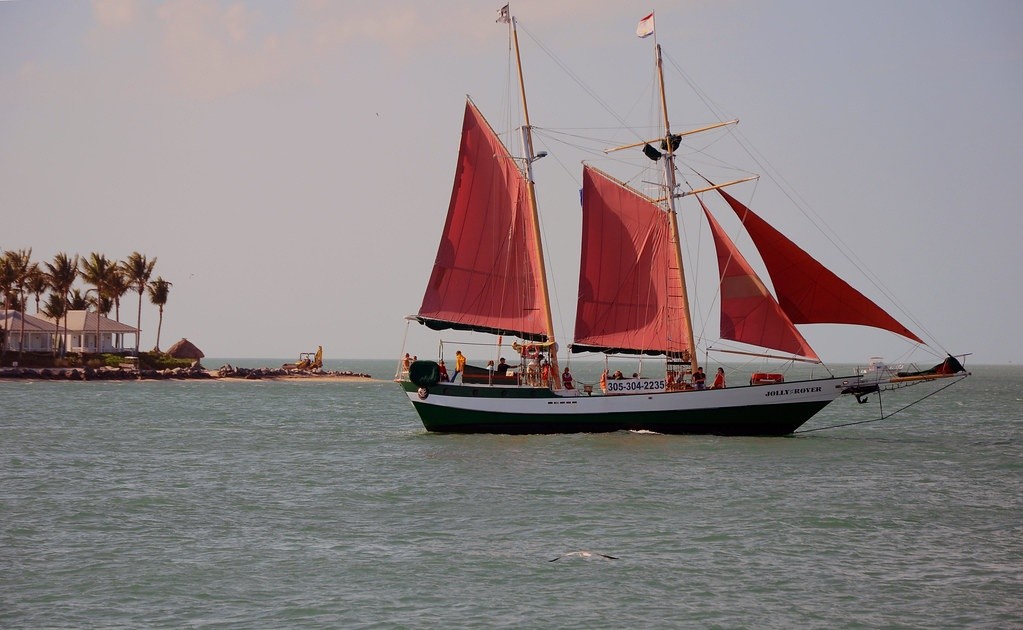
[713,367,725,388]
[405,353,417,369]
[497,358,521,375]
[562,367,576,390]
[439,361,450,383]
[612,371,623,379]
[485,361,494,371]
[692,366,706,390]
[526,354,544,374]
[449,351,465,382]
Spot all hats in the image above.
[500,358,506,360]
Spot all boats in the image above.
[850,356,906,380]
[281,346,323,370]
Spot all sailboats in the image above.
[395,3,975,437]
